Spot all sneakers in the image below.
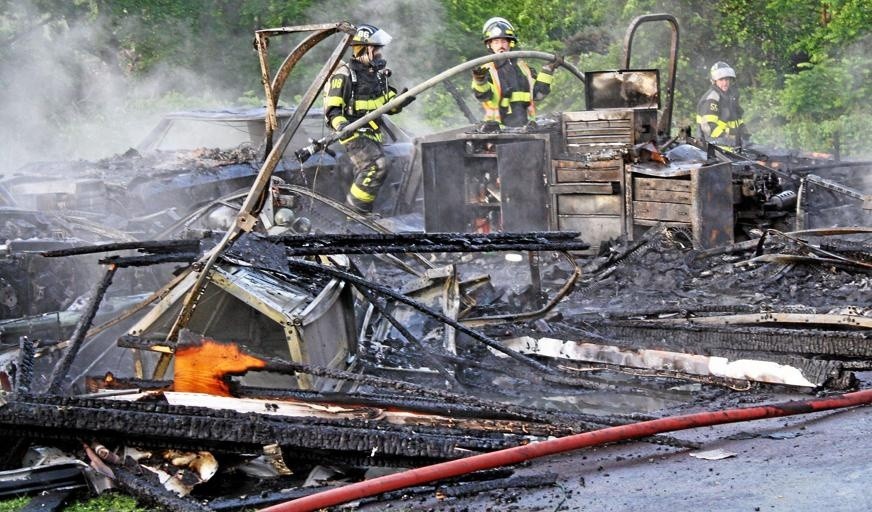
[346,212,383,222]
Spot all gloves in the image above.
[547,53,564,71]
[393,88,416,109]
[471,66,490,81]
[337,123,356,141]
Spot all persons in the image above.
[322,24,418,225]
[692,61,753,152]
[467,16,564,129]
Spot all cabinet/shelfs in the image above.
[549,158,735,250]
[419,131,563,232]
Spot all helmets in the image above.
[479,17,518,54]
[710,61,736,81]
[348,24,392,46]
